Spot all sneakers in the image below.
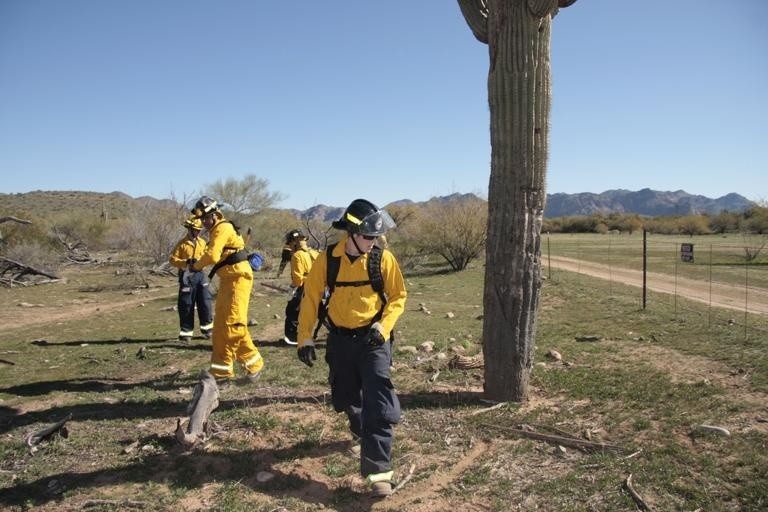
[245,371,261,382]
[181,336,191,342]
[215,377,231,394]
[205,330,212,338]
[350,440,360,459]
[372,482,392,497]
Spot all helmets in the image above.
[332,199,396,237]
[190,195,223,219]
[180,215,203,230]
[284,228,308,245]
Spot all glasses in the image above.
[363,235,378,240]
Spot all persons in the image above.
[297,199,406,498]
[169,214,213,343]
[280,230,320,347]
[277,230,295,280]
[189,196,264,385]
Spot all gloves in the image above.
[186,258,200,273]
[363,328,385,346]
[297,346,317,367]
